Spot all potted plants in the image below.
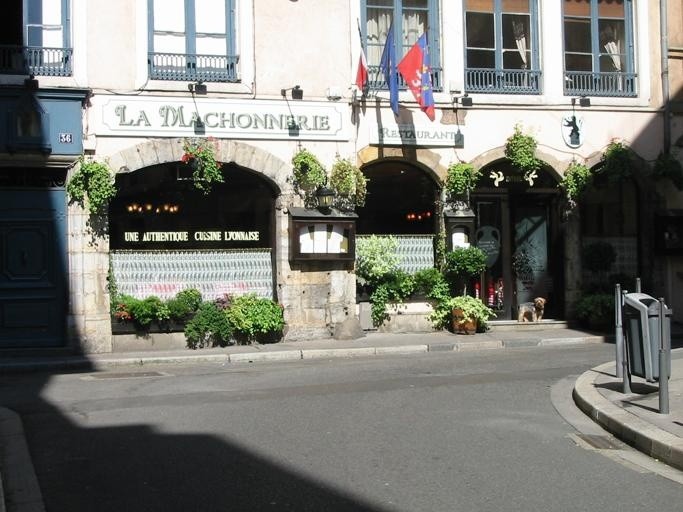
[292,149,329,205]
[333,158,368,213]
[503,132,543,172]
[67,160,117,214]
[595,143,650,184]
[445,162,481,201]
[558,162,593,208]
[428,295,498,335]
[183,139,226,194]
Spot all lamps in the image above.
[315,186,334,215]
[281,86,303,100]
[188,79,207,95]
[571,93,591,107]
[453,93,473,106]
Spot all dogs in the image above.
[516,296,548,323]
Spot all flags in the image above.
[353,28,367,92]
[397,30,436,122]
[381,18,400,116]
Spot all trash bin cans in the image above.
[624,292,673,379]
